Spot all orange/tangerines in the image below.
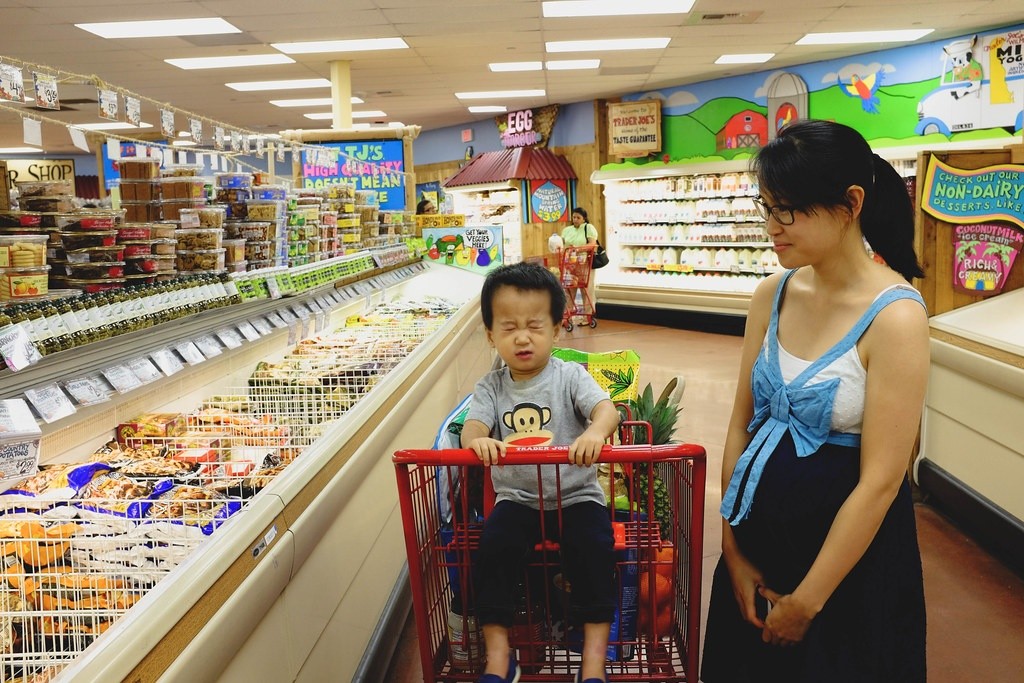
[634,539,679,638]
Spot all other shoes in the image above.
[574,664,610,683]
[474,648,522,683]
[577,320,589,327]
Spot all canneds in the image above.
[445,595,548,674]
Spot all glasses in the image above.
[752,195,817,225]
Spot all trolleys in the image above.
[524,244,597,332]
[391,404,707,683]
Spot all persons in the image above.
[460,261,620,683]
[416,200,436,215]
[556,207,598,326]
[702,119,928,683]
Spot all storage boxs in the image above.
[546,546,643,663]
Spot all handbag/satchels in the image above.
[584,222,610,269]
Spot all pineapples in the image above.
[614,379,686,542]
